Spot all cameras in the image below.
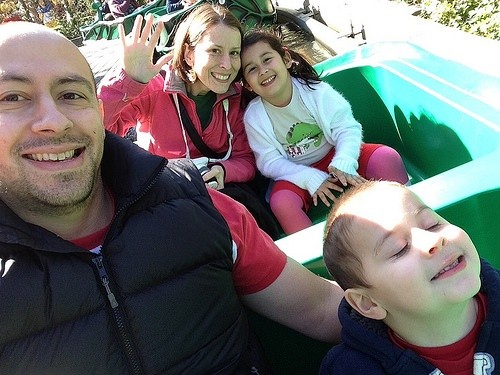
[188,156,219,190]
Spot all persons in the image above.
[94,1,208,20]
[318,180,499,375]
[96,4,280,241]
[237,32,410,236]
[1,17,345,375]
